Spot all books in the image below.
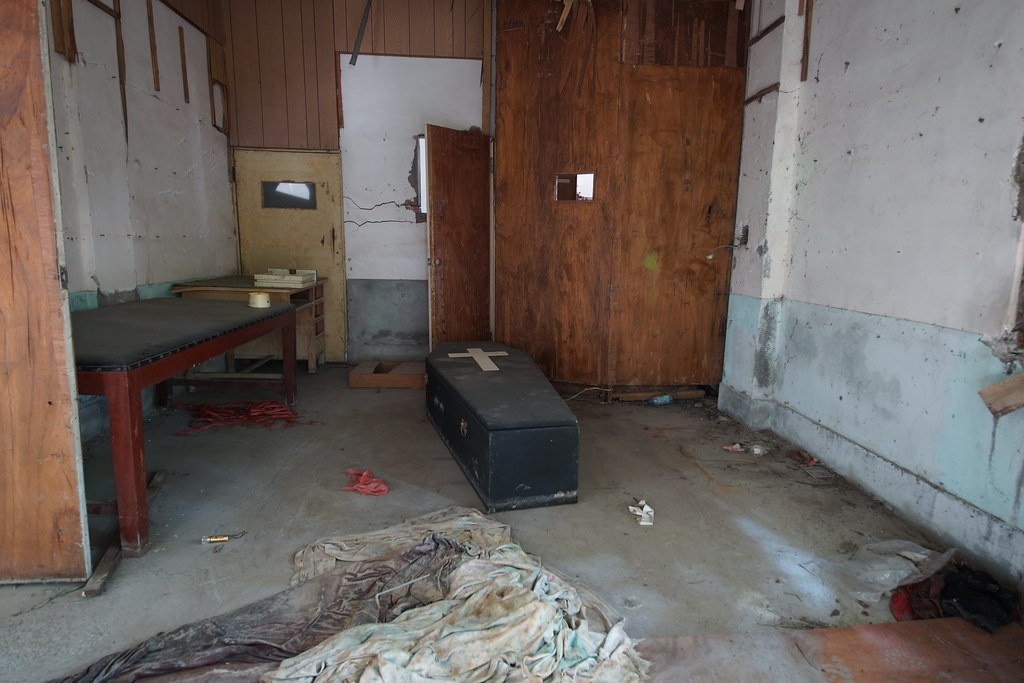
[254,267,317,288]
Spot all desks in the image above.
[173,275,328,376]
[69,296,299,559]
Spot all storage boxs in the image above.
[427,338,581,511]
